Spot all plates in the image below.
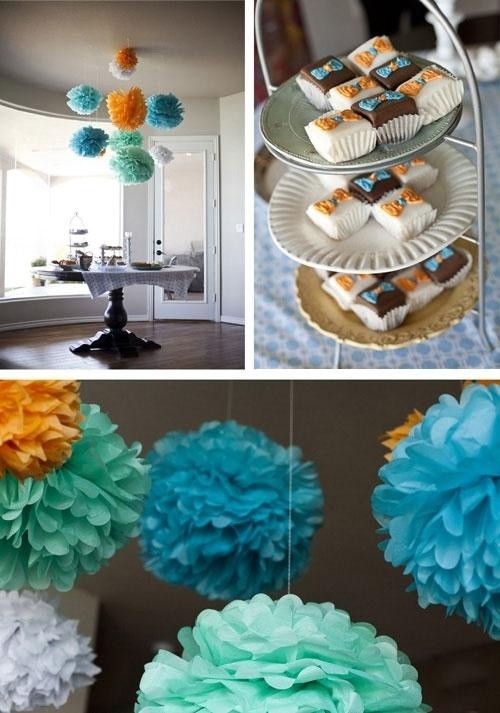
[99,266,128,272]
[266,144,480,275]
[259,54,465,175]
[293,236,481,352]
[132,265,162,271]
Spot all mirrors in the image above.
[160,149,208,305]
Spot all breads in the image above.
[369,55,421,90]
[315,268,335,281]
[306,187,371,240]
[347,35,398,75]
[395,68,465,126]
[328,75,386,110]
[295,55,356,111]
[388,158,439,193]
[422,245,471,288]
[372,186,438,240]
[304,107,377,164]
[350,278,412,329]
[351,90,427,145]
[391,266,444,314]
[348,169,402,206]
[321,272,378,311]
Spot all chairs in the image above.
[29,258,201,370]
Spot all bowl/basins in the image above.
[60,264,75,271]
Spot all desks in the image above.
[252,45,500,370]
